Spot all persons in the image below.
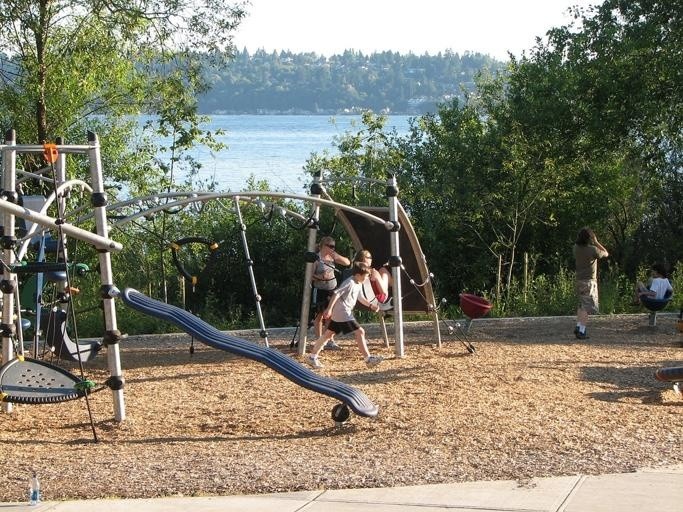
[306,237,392,369]
[628,264,674,306]
[573,228,609,339]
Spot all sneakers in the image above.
[319,335,342,351]
[305,354,325,369]
[573,325,590,340]
[365,355,384,369]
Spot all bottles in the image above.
[30,473,40,506]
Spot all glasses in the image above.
[325,244,335,249]
[365,256,372,259]
